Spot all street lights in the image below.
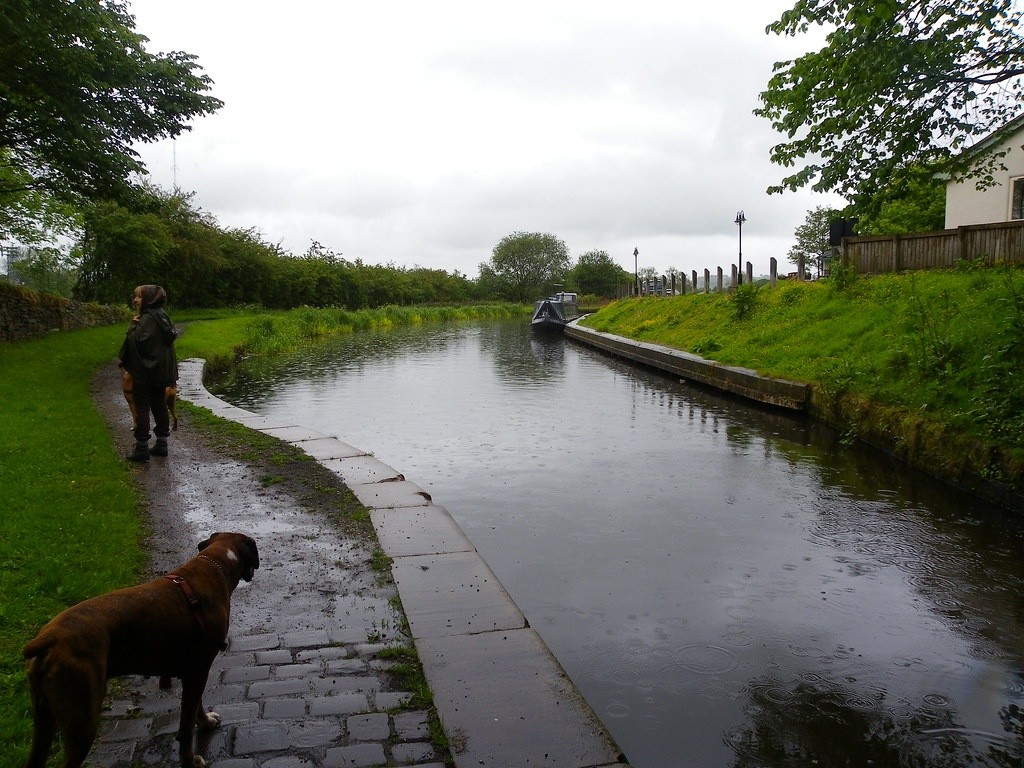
[733,209,746,286]
[633,247,639,296]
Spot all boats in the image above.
[532,291,580,325]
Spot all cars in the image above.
[642,280,662,291]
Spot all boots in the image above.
[126,440,150,460]
[149,436,168,457]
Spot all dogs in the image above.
[20,531,261,768]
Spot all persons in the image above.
[121,285,175,461]
